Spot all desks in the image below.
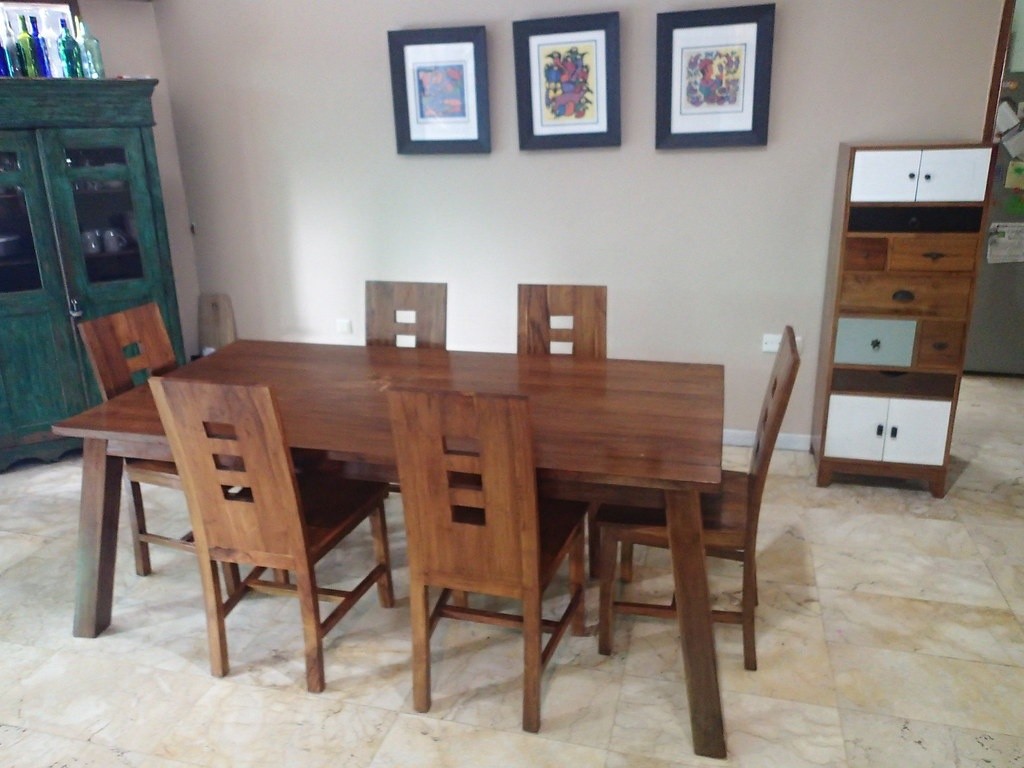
[52,338,725,759]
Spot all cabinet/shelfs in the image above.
[808,134,999,497]
[0,78,189,475]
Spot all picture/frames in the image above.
[386,24,491,156]
[0,0,81,78]
[655,3,777,149]
[512,10,622,152]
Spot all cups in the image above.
[81,232,101,253]
[103,230,127,253]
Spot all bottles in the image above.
[17,15,38,78]
[0,42,9,77]
[74,15,104,79]
[29,16,52,78]
[2,11,26,78]
[56,19,83,78]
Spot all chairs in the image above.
[51,281,802,735]
